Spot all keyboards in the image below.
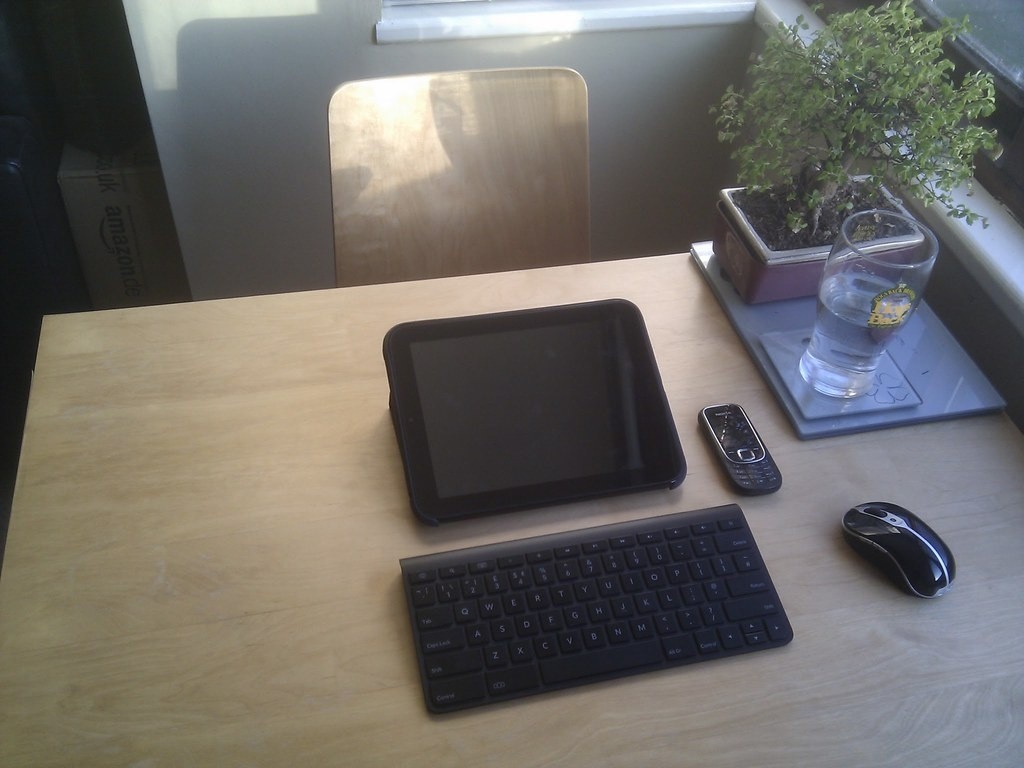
[400,504,795,713]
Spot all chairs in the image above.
[328,68,591,292]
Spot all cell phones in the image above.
[697,403,783,496]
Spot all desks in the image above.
[0,237,1024,768]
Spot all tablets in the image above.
[383,298,688,524]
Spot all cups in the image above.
[799,209,939,399]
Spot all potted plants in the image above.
[710,0,1005,305]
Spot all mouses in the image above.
[844,502,957,599]
